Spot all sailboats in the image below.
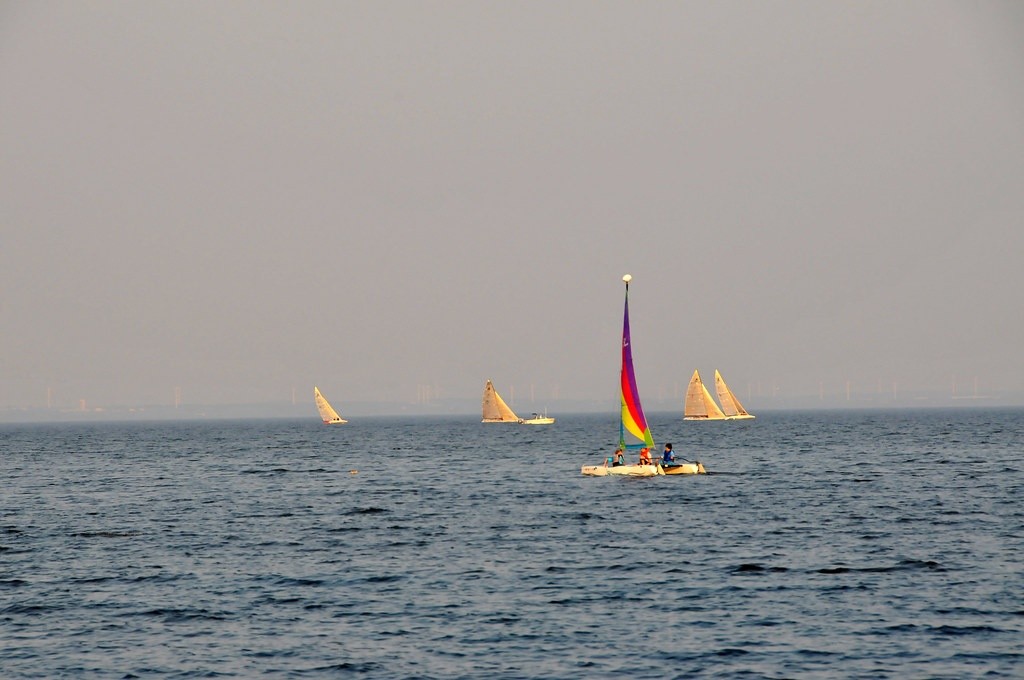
[481,380,524,424]
[713,370,756,421]
[314,385,348,424]
[682,369,727,421]
[578,273,706,476]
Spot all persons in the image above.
[658,443,676,465]
[612,449,625,466]
[637,447,652,465]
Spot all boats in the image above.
[525,415,554,424]
